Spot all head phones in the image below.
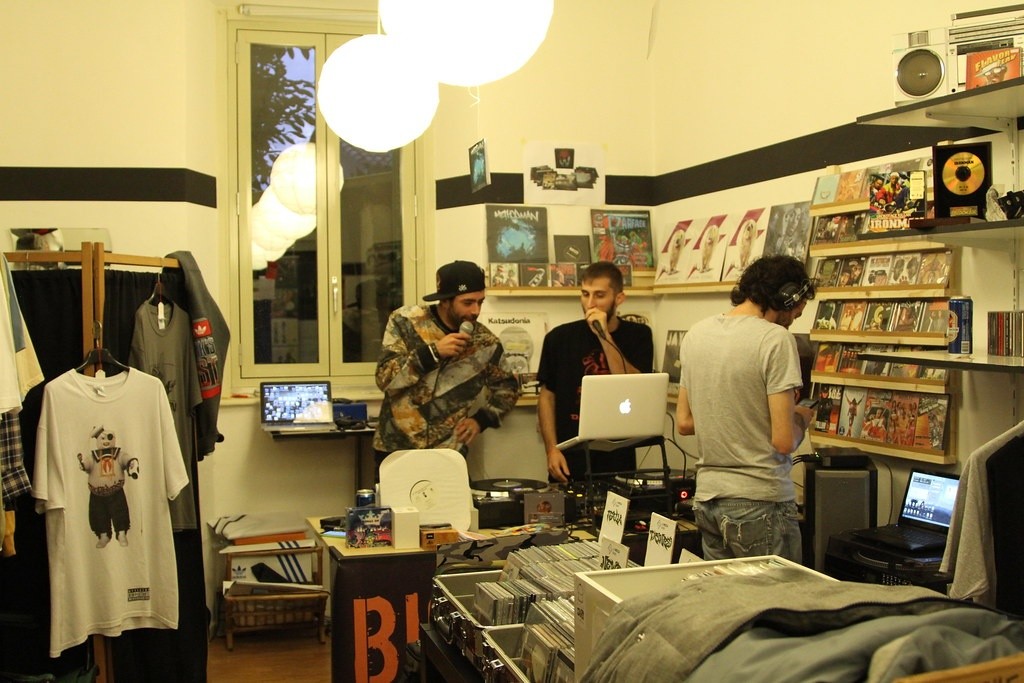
[776,280,811,311]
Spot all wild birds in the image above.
[556,271,565,285]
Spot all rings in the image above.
[467,429,472,435]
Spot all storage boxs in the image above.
[430,555,841,683]
[209,532,329,650]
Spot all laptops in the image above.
[556,373,669,452]
[260,381,338,432]
[848,466,962,552]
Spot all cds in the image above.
[943,152,985,194]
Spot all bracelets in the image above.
[431,343,440,358]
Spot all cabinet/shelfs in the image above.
[485,75,1024,465]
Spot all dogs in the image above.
[729,219,756,271]
[663,230,686,274]
[693,224,719,272]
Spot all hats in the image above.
[420,261,487,302]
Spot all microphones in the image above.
[592,319,606,340]
[439,321,474,370]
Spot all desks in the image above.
[270,422,379,508]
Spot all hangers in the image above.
[75,319,130,373]
[147,256,173,304]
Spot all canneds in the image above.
[356,489,376,508]
[947,295,974,358]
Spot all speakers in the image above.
[893,44,958,106]
[803,462,878,574]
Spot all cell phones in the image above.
[797,398,819,409]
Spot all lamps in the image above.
[379,0,555,87]
[316,0,441,153]
[252,143,345,271]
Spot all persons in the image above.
[845,394,864,436]
[816,386,833,432]
[817,216,863,239]
[675,254,816,559]
[529,267,564,286]
[838,263,862,287]
[536,260,654,483]
[493,265,518,287]
[345,280,396,361]
[841,305,863,331]
[925,310,943,331]
[817,302,836,329]
[372,260,518,484]
[876,400,917,445]
[869,172,923,218]
[840,350,865,369]
